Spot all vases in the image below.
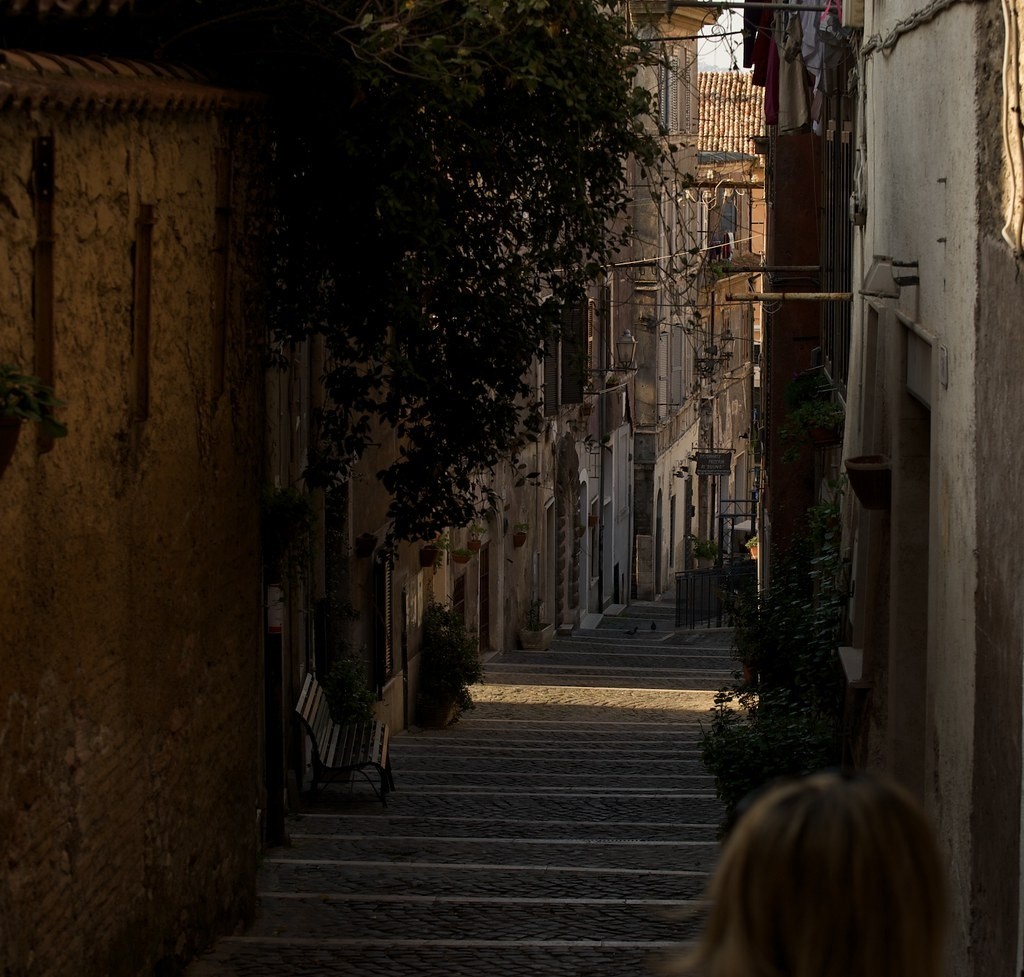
[356,531,377,557]
[844,455,889,508]
[589,517,597,527]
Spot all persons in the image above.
[657,773,946,977]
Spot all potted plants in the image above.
[466,524,488,552]
[518,599,553,650]
[419,532,451,568]
[686,535,719,569]
[512,524,528,547]
[418,602,480,729]
[579,525,587,537]
[745,536,758,557]
[778,401,844,462]
[810,497,841,529]
[451,549,472,563]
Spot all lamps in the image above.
[858,257,918,301]
[579,328,637,374]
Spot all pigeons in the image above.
[651,619,657,633]
[623,627,638,638]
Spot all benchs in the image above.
[294,673,396,808]
